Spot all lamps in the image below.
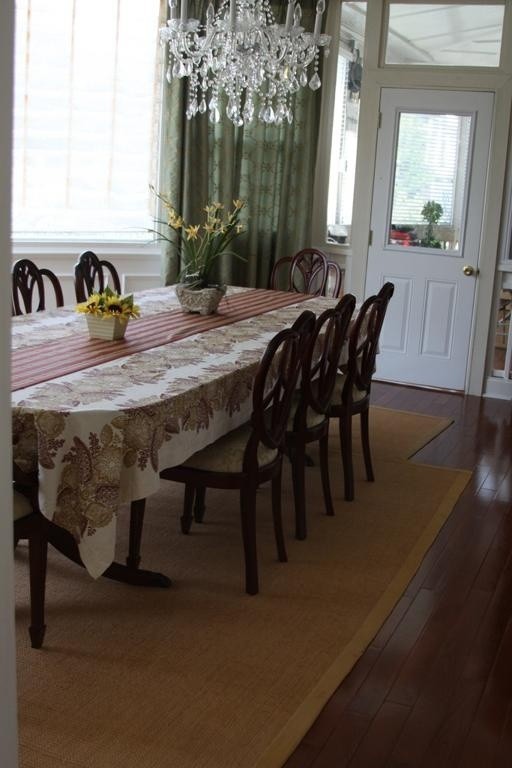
[155,0,334,131]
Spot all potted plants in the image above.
[421,199,444,242]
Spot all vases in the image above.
[175,287,227,316]
[84,315,129,340]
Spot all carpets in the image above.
[12,404,475,766]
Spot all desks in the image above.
[9,284,377,647]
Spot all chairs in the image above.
[124,280,393,595]
[269,249,344,298]
[74,251,121,303]
[9,258,65,315]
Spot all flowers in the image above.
[77,286,142,320]
[132,181,250,287]
